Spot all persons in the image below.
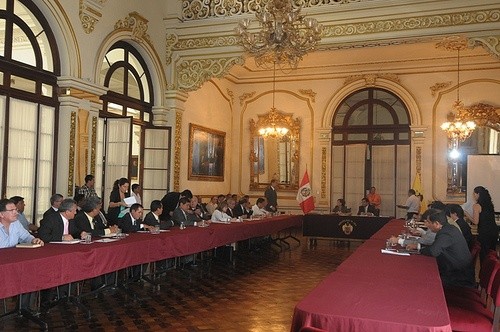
[386,200,473,254]
[187,196,208,220]
[406,212,476,306]
[117,203,155,285]
[170,198,209,271]
[251,198,273,250]
[107,178,129,226]
[0,199,44,318]
[38,174,118,307]
[205,193,253,259]
[465,186,499,290]
[131,184,142,205]
[158,189,193,221]
[265,179,279,213]
[10,196,39,237]
[397,189,421,220]
[357,198,375,216]
[143,200,176,277]
[368,187,380,209]
[332,199,347,213]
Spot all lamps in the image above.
[257,62,289,143]
[234,0,320,72]
[441,49,476,142]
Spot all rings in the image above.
[388,240,389,241]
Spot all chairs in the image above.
[447,241,500,332]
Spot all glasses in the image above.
[5,207,18,213]
[55,200,63,203]
[70,209,78,213]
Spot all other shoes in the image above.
[157,267,166,275]
[53,294,59,305]
[128,277,144,285]
[475,278,485,291]
[37,294,50,307]
[136,275,152,283]
[15,303,29,315]
[59,285,69,299]
[90,282,106,292]
[185,262,197,267]
[175,266,182,271]
[115,278,125,288]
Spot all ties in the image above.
[64,221,69,235]
[134,221,136,226]
[92,218,97,230]
[100,212,108,224]
[274,190,277,206]
[194,208,206,220]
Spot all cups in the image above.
[201,220,205,227]
[181,222,185,230]
[155,225,160,234]
[85,233,91,244]
[227,218,231,224]
[250,215,253,221]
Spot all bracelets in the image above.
[117,202,120,207]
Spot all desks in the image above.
[291,218,452,332]
[0,215,303,332]
[303,214,389,249]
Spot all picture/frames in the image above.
[188,123,227,182]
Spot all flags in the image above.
[295,170,315,216]
[412,170,426,214]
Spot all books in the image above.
[123,196,137,208]
[15,243,41,248]
